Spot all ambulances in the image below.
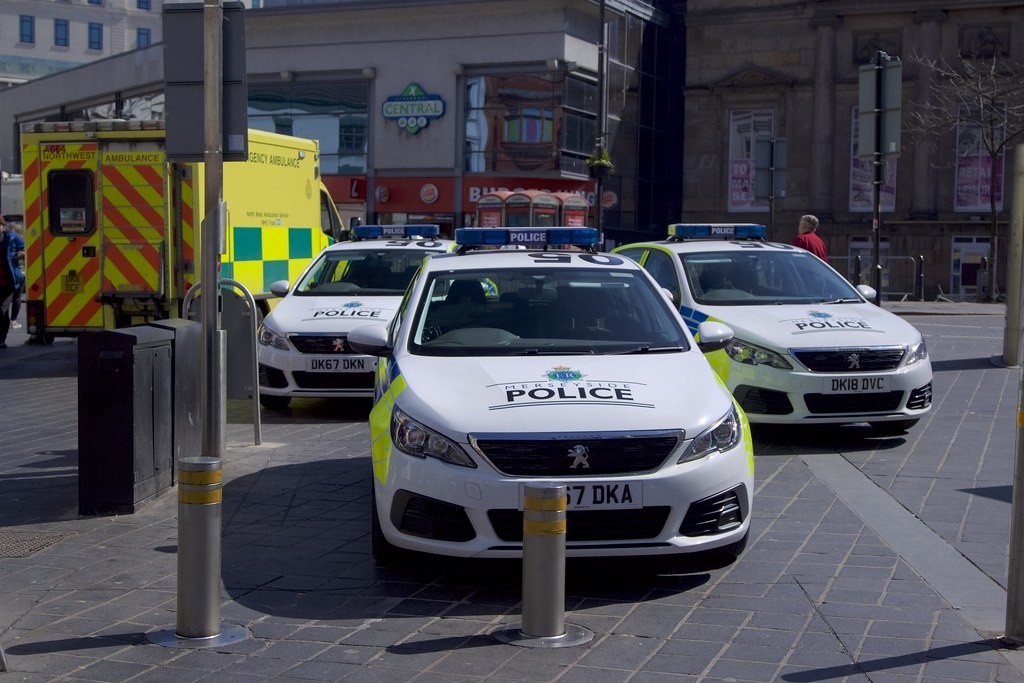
[16,121,363,337]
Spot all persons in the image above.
[424,280,483,341]
[791,215,829,264]
[0,214,26,348]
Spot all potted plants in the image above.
[584,145,616,178]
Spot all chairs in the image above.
[429,280,488,329]
[699,270,732,295]
[350,265,419,290]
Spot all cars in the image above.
[257,223,462,411]
[345,225,756,580]
[609,225,933,435]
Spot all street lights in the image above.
[871,51,902,304]
